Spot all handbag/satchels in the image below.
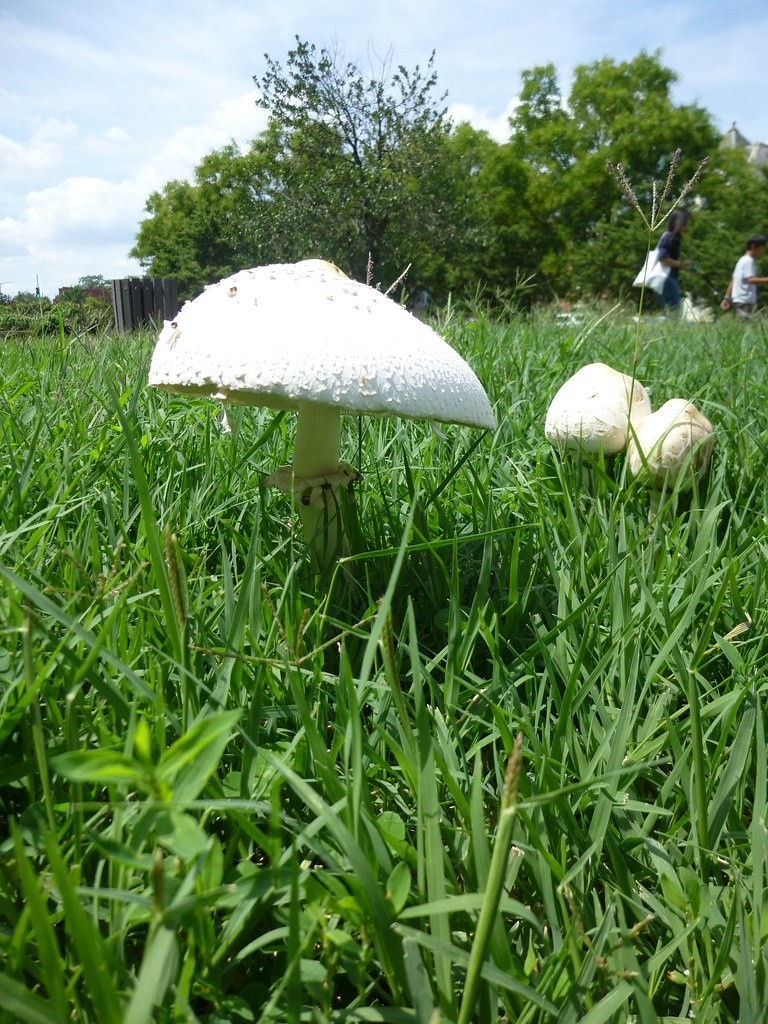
[632,231,671,295]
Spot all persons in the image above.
[720,235,768,314]
[657,210,695,310]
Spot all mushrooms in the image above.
[148,259,496,581]
[544,362,713,524]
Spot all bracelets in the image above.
[724,296,731,302]
